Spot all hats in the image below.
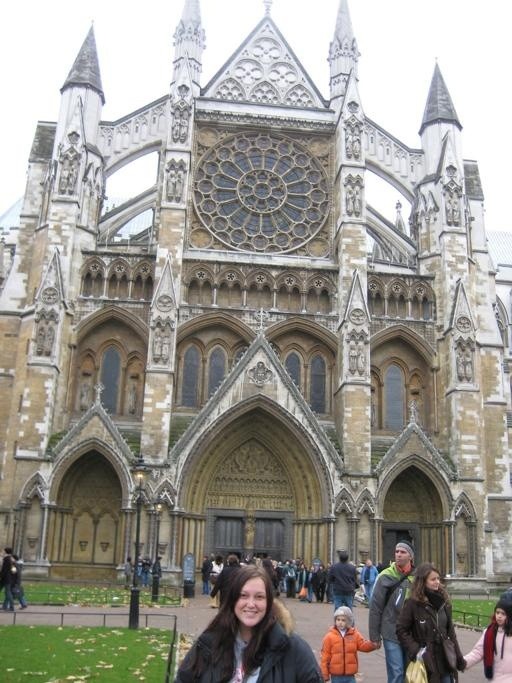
[494,592,512,612]
[396,539,416,560]
[333,604,353,621]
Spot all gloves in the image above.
[455,656,467,669]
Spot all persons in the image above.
[322,606,379,683]
[173,565,325,683]
[458,587,512,683]
[395,563,466,683]
[2,556,29,610]
[369,540,418,683]
[198,553,380,625]
[122,553,163,590]
[0,548,16,612]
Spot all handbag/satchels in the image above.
[403,659,430,682]
[433,621,459,674]
[299,585,308,596]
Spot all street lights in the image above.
[129,449,152,630]
[148,498,168,601]
